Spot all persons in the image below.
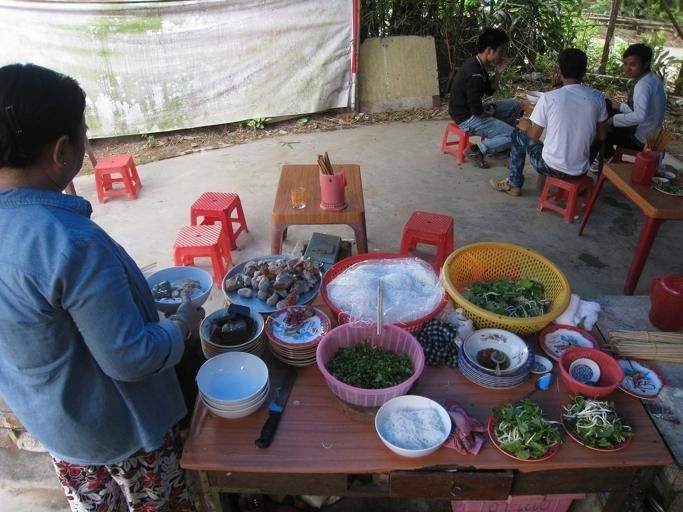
[0,62,204,512]
[491,47,609,195]
[449,27,521,167]
[590,43,665,173]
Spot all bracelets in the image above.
[169,315,192,341]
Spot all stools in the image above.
[439,122,486,166]
[399,210,456,269]
[93,151,144,205]
[189,190,249,252]
[615,144,640,164]
[536,171,594,224]
[171,225,234,290]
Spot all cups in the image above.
[317,168,347,212]
[289,188,305,210]
[656,162,665,177]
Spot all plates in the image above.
[662,171,675,179]
[560,401,633,452]
[530,354,553,375]
[222,253,324,314]
[264,306,330,367]
[650,177,682,197]
[538,323,599,363]
[487,415,559,461]
[611,355,669,400]
[457,339,535,391]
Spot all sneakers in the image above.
[488,177,522,197]
[589,151,622,173]
[462,143,491,169]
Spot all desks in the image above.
[177,294,676,511]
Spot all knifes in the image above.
[254,367,299,448]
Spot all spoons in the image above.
[513,372,554,404]
[490,350,505,376]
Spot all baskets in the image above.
[315,320,426,409]
[319,251,450,335]
[439,240,572,339]
[557,345,624,402]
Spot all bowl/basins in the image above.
[559,347,623,398]
[195,350,270,421]
[146,267,214,312]
[198,309,264,359]
[463,328,529,374]
[526,91,544,105]
[373,394,452,458]
[569,358,600,384]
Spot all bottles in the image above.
[632,151,657,185]
[648,275,682,332]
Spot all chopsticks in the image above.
[375,279,384,336]
[645,128,673,152]
[316,152,334,175]
